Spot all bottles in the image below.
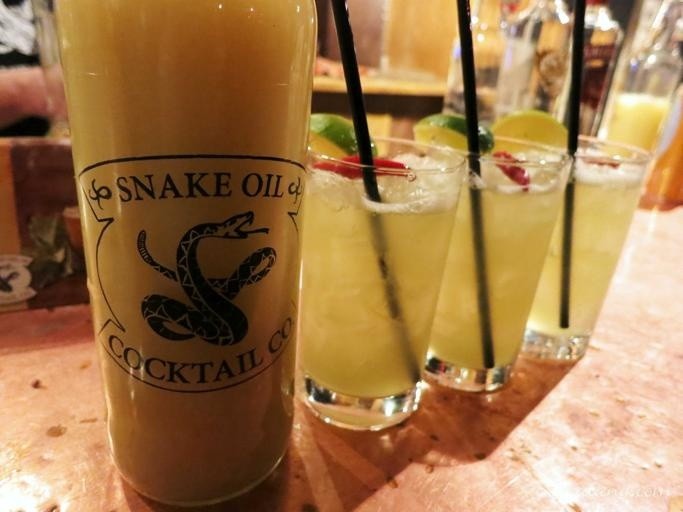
[604,0,683,150]
[494,1,576,122]
[557,1,626,136]
[444,1,512,134]
[638,84,682,211]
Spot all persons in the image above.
[0,1,65,136]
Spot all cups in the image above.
[294,138,466,434]
[496,133,652,364]
[46,0,319,510]
[405,135,573,394]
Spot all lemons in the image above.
[308,112,378,159]
[412,113,495,155]
[491,109,570,149]
